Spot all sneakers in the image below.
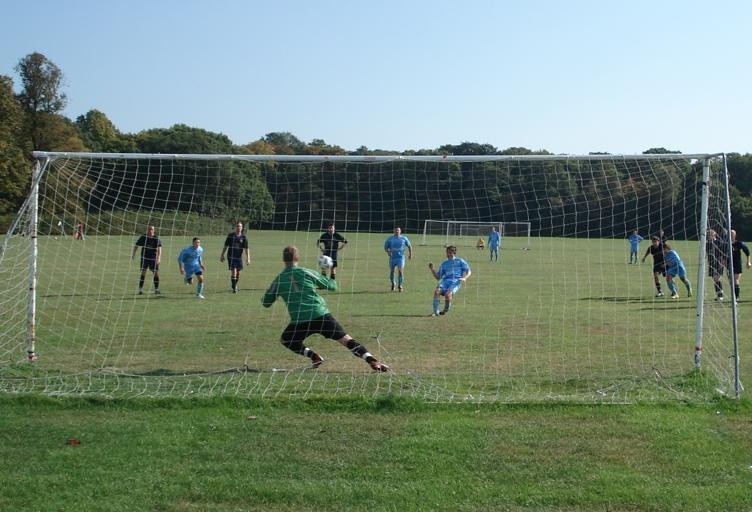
[688,289,692,297]
[156,289,160,294]
[309,357,325,368]
[398,284,403,289]
[656,292,664,297]
[369,361,389,372]
[431,312,439,316]
[391,282,396,290]
[233,288,238,293]
[670,294,679,298]
[715,296,723,301]
[196,293,205,299]
[439,310,448,315]
[184,277,189,286]
[139,291,143,294]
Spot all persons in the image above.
[487,226,501,262]
[220,221,251,294]
[73,221,85,240]
[261,246,390,373]
[131,225,162,295]
[642,229,751,304]
[178,238,207,300]
[384,226,413,292]
[316,224,348,280]
[476,237,485,250]
[428,246,472,317]
[627,228,643,264]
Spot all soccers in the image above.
[318,256,334,271]
